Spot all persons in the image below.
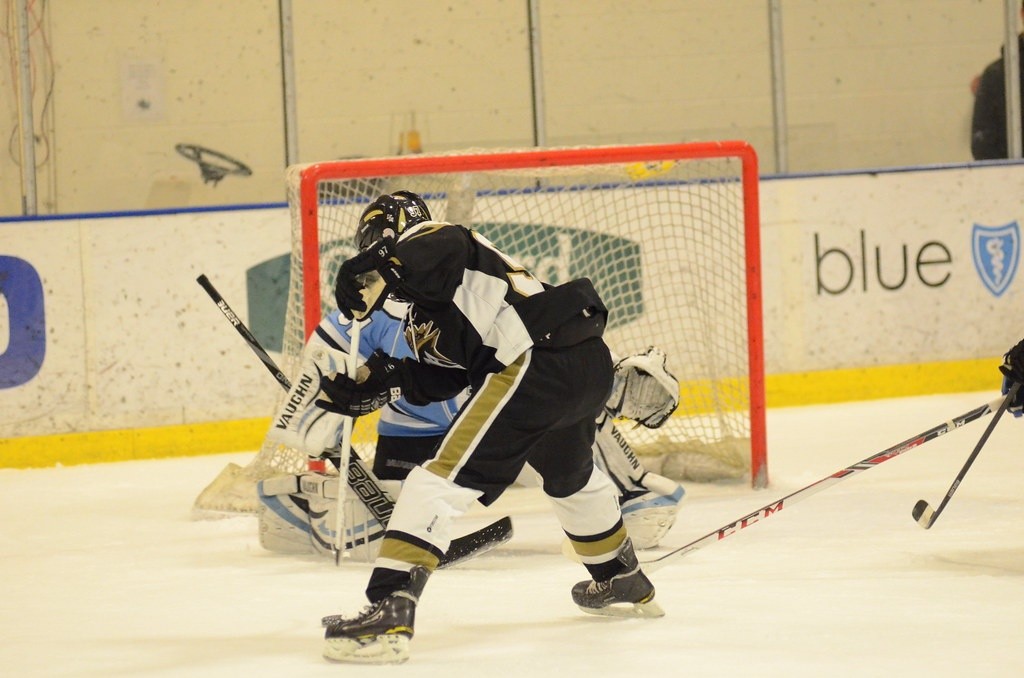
[267,266,679,564]
[322,190,656,638]
[1001,333,1024,418]
[968,0,1024,171]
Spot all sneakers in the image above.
[572,539,664,620]
[318,582,414,664]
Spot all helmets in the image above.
[353,188,432,255]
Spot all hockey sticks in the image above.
[910,377,1020,530]
[638,389,1018,576]
[196,272,516,571]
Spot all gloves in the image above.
[316,363,403,418]
[998,338,1024,418]
[306,454,340,479]
[336,250,379,322]
